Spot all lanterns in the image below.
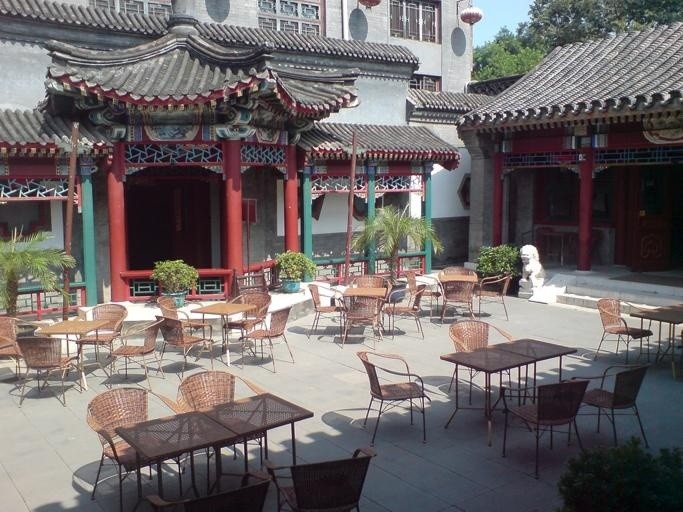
[358,0,381,10]
[459,4,484,26]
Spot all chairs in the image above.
[535,227,566,264]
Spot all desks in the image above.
[629,305,682,382]
[543,232,576,266]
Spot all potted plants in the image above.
[152,259,200,309]
[270,251,317,290]
[474,242,521,295]
[349,202,443,303]
